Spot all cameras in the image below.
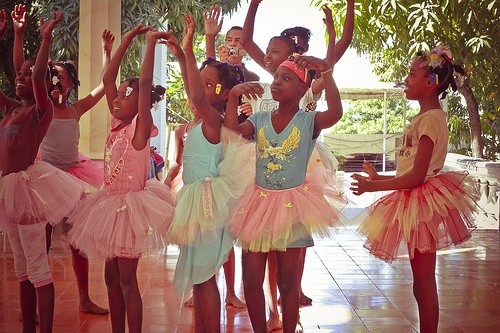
[227,47,247,57]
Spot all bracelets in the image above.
[41,35,51,41]
[321,67,332,74]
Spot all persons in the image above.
[350,44,481,333]
[163,95,254,308]
[11,4,115,326]
[157,12,245,333]
[0,6,87,333]
[202,3,336,333]
[65,22,178,333]
[222,24,262,82]
[224,54,346,333]
[240,0,355,307]
